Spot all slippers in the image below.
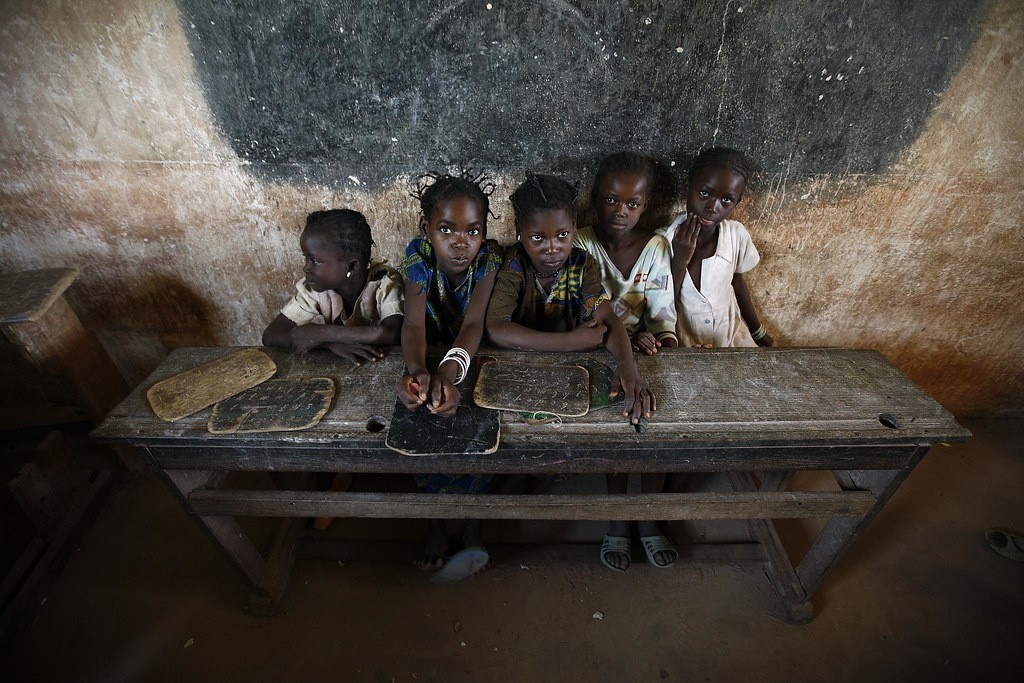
[639,534,679,568]
[600,532,632,573]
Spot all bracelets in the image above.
[439,348,470,384]
[753,325,766,340]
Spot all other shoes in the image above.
[433,545,488,587]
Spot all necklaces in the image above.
[537,272,558,278]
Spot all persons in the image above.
[655,147,773,349]
[262,207,407,529]
[397,165,503,587]
[573,153,680,573]
[485,174,656,529]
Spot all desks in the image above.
[91,347,975,622]
[0,266,150,476]
